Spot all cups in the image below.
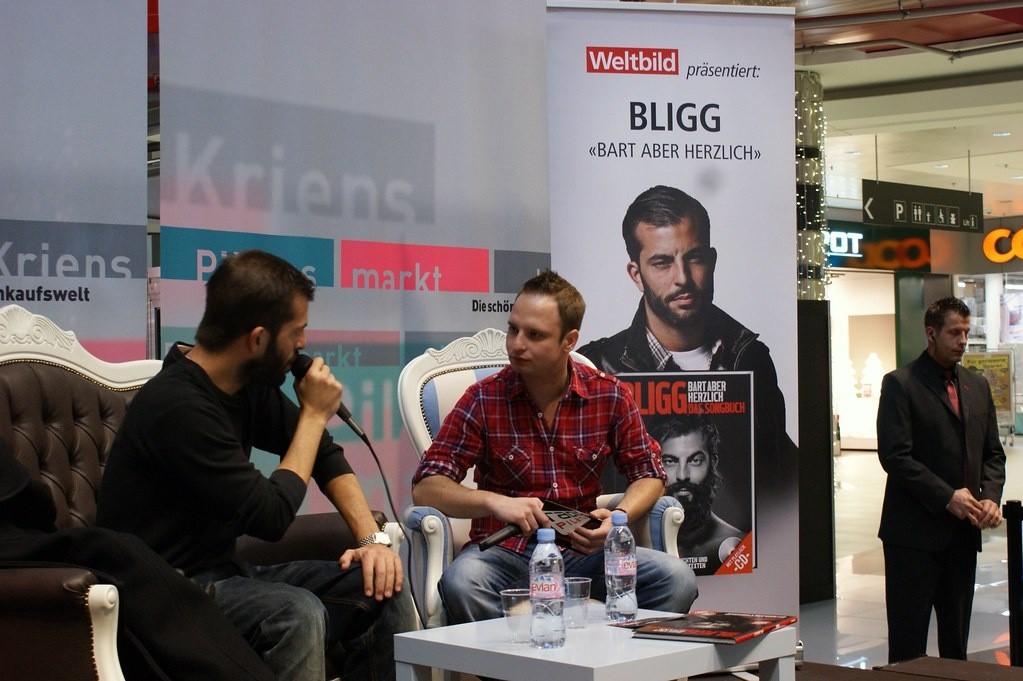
[563,577,592,628]
[500,589,531,643]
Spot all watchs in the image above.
[358,532,392,548]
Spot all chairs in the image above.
[398,328,598,681]
[0,303,163,681]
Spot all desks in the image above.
[393,604,797,681]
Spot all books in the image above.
[632,610,797,645]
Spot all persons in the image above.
[0,438,278,681]
[576,186,798,506]
[97,249,417,681]
[648,415,746,575]
[876,298,1006,666]
[410,271,699,626]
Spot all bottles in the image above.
[604,514,638,621]
[528,528,565,648]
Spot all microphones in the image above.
[477,523,523,553]
[289,353,370,444]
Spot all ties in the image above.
[947,373,959,417]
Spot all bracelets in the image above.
[610,508,626,513]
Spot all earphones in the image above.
[929,332,935,342]
